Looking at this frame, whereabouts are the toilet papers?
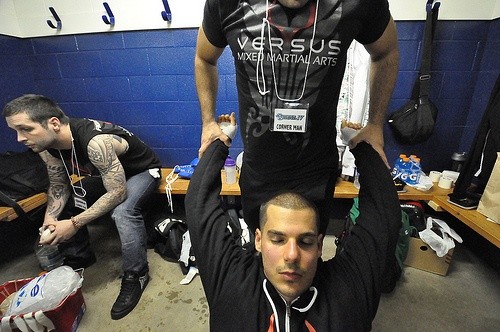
[428,171,441,182]
[438,177,453,189]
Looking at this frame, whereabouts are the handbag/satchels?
[148,212,187,262]
[477,151,500,224]
[0,149,50,203]
[388,95,438,145]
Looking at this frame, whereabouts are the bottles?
[394,154,421,184]
[224,155,236,184]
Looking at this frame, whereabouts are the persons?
[195,1,400,234]
[184,111,400,332]
[3,95,162,319]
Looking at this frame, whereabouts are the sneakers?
[62,253,97,272]
[110,264,150,320]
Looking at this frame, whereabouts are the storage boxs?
[0,277,88,332]
[403,237,456,277]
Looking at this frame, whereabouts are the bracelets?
[71,216,80,230]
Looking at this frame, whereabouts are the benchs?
[432,194,500,248]
[154,168,242,211]
[0,173,86,222]
[332,175,456,200]
